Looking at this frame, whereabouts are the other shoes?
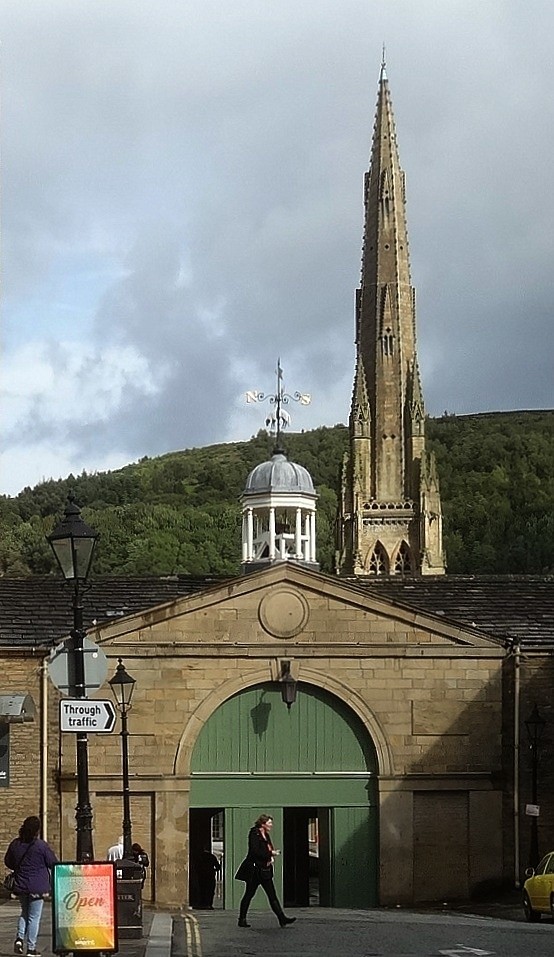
[208,906,214,910]
[279,917,296,928]
[202,906,207,910]
[237,918,251,928]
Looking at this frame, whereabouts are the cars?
[521,852,554,922]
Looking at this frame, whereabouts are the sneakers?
[14,940,23,954]
[26,949,41,957]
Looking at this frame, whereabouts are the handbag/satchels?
[245,856,267,886]
[4,875,15,891]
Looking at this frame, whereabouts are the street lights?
[107,657,139,862]
[45,494,101,865]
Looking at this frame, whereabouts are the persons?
[131,842,149,890]
[4,816,61,957]
[233,815,297,928]
[195,845,221,910]
[107,835,124,879]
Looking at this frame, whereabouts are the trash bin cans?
[116,857,143,938]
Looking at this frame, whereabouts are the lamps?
[280,660,297,711]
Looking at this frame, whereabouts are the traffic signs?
[60,698,116,734]
